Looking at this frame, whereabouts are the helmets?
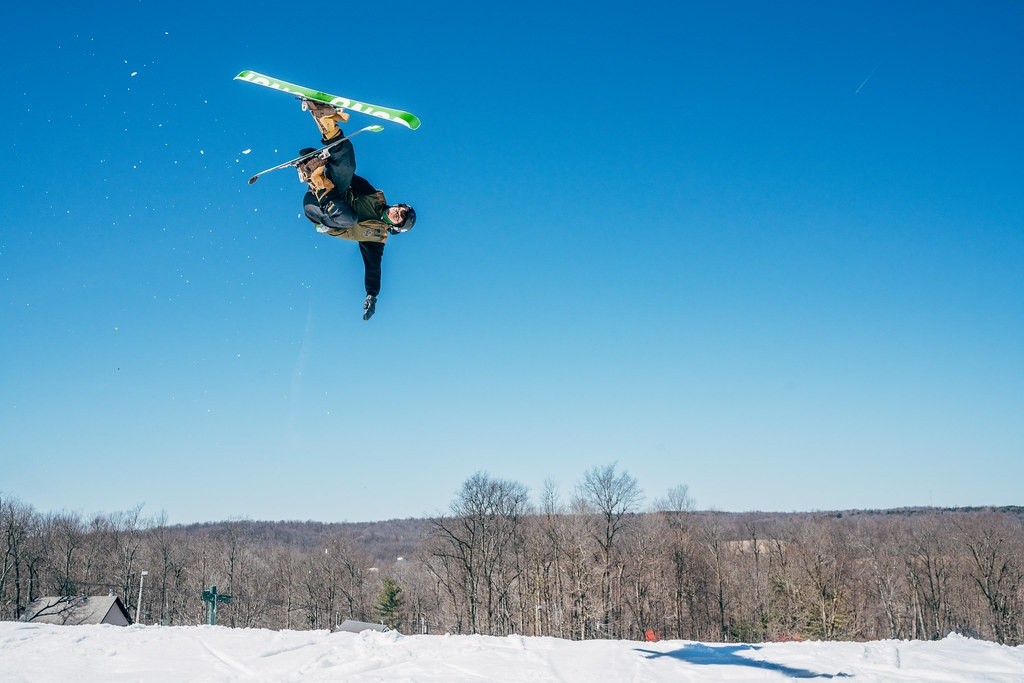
[388,203,416,233]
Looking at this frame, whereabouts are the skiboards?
[232,71,421,185]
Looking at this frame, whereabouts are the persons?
[293,98,417,320]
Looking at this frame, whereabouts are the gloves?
[300,148,317,155]
[363,295,377,321]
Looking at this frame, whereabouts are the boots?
[302,159,335,201]
[309,109,350,140]
